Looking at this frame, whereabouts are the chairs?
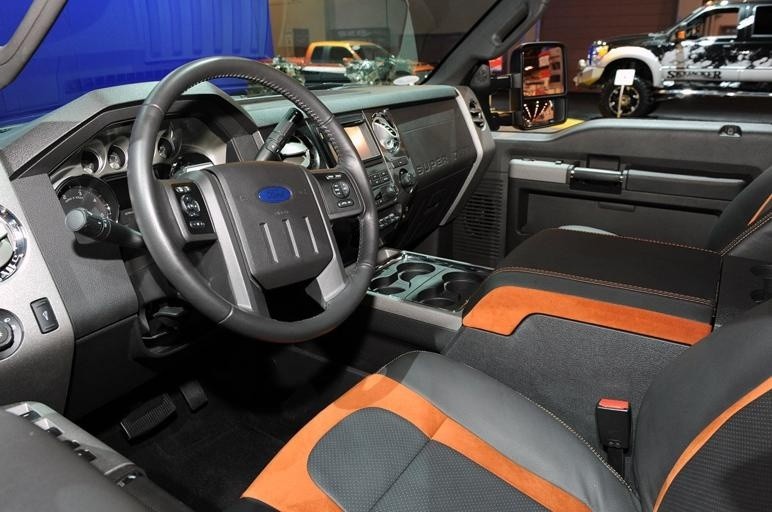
[219,298,771,511]
[462,173,771,347]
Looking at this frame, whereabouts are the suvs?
[572,0,772,118]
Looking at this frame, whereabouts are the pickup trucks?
[254,40,435,85]
